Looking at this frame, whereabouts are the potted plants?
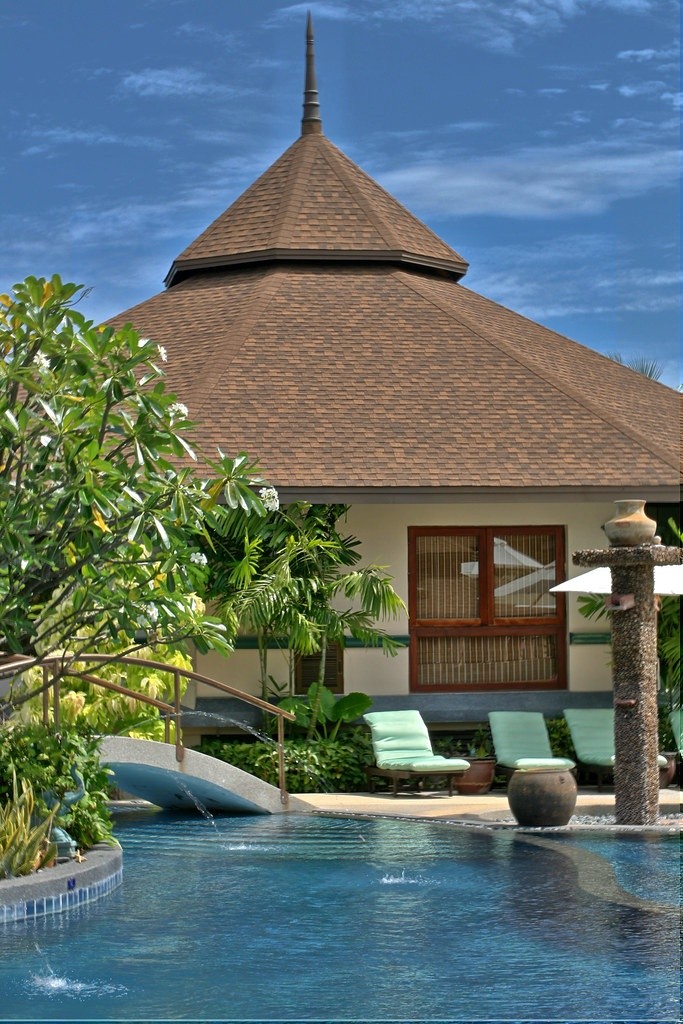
[449,744,497,795]
[658,752,677,789]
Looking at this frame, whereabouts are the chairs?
[353,709,471,797]
[488,711,576,793]
[563,708,667,792]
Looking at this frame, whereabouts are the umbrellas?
[547,548,683,596]
[460,535,557,607]
